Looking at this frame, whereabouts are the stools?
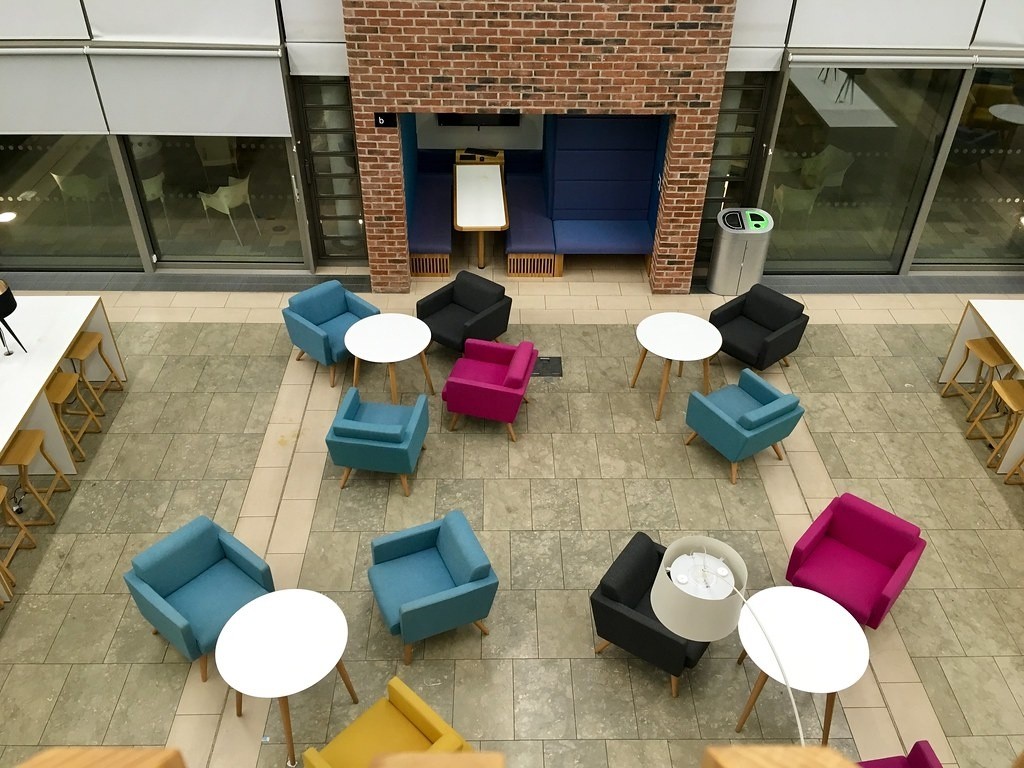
[47,330,124,462]
[777,88,820,148]
[0,429,72,587]
[941,337,1024,486]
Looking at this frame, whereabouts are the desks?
[0,296,127,601]
[769,148,804,175]
[631,312,723,420]
[790,68,899,205]
[103,137,162,160]
[344,313,435,403]
[736,585,869,746]
[215,588,358,766]
[937,299,1024,475]
[986,103,1024,172]
[453,163,509,268]
[735,585,870,746]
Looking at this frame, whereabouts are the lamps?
[651,536,806,747]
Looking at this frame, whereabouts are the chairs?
[590,531,710,698]
[122,515,275,681]
[961,83,1020,140]
[302,675,474,768]
[282,280,380,386]
[708,283,809,373]
[141,172,172,236]
[685,368,804,483]
[769,181,825,229]
[442,337,539,441]
[786,493,927,632]
[857,740,944,768]
[416,269,512,357]
[194,136,240,192]
[325,387,430,497]
[798,144,856,206]
[199,172,262,247]
[50,171,115,226]
[367,507,500,666]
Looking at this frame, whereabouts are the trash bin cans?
[705,207,775,296]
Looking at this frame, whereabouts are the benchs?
[552,220,654,278]
[506,174,554,277]
[408,174,452,277]
